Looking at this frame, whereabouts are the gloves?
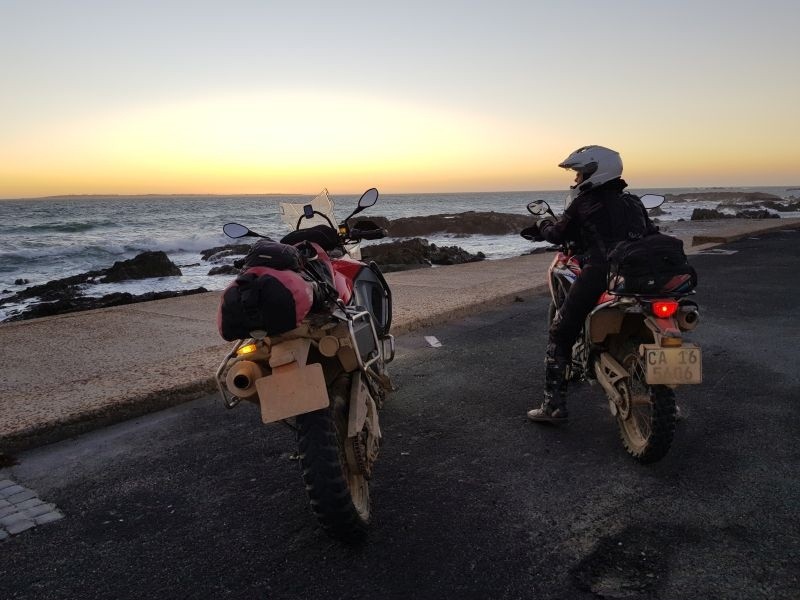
[520,219,554,242]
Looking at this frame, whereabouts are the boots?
[675,405,680,421]
[527,356,572,423]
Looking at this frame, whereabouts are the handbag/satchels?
[217,240,335,342]
[605,220,698,293]
[245,238,305,272]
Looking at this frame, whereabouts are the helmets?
[558,145,623,202]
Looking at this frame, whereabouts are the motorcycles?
[216,187,398,533]
[524,194,702,463]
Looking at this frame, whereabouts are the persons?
[528,145,660,422]
